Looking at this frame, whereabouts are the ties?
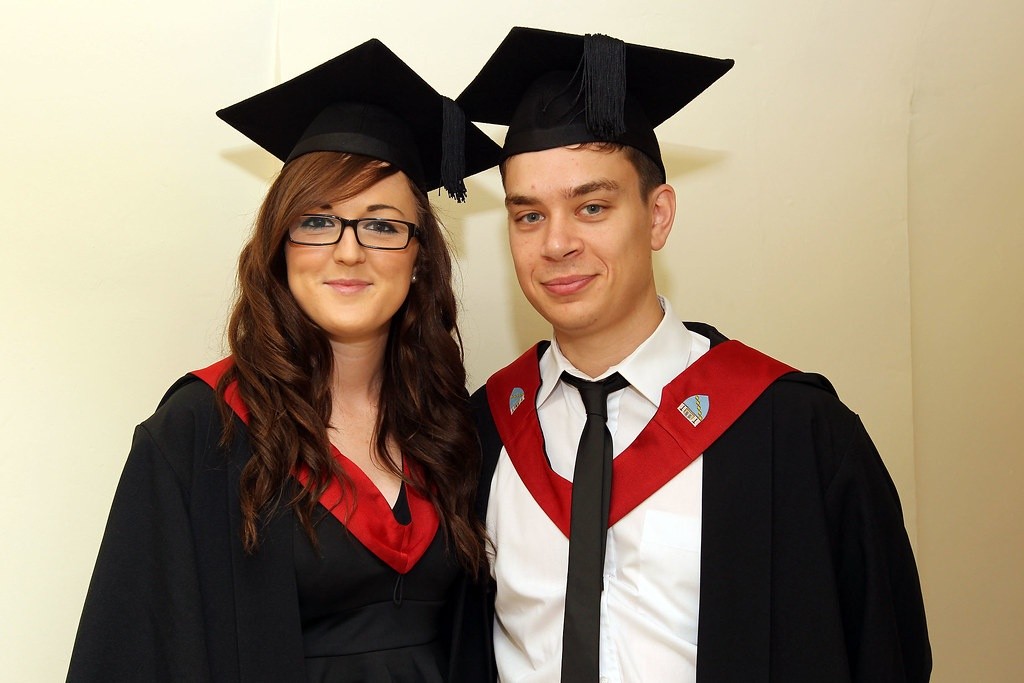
[560,370,631,683]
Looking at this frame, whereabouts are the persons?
[66,36,503,683]
[454,26,932,683]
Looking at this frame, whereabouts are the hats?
[456,26,735,185]
[216,38,503,199]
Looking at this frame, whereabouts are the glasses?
[287,214,425,250]
